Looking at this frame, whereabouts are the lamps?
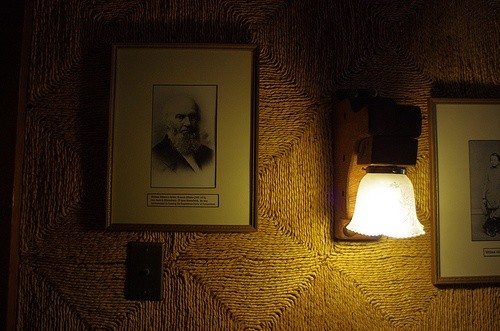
[331,88,426,247]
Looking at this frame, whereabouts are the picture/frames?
[102,40,262,234]
[426,94,500,289]
